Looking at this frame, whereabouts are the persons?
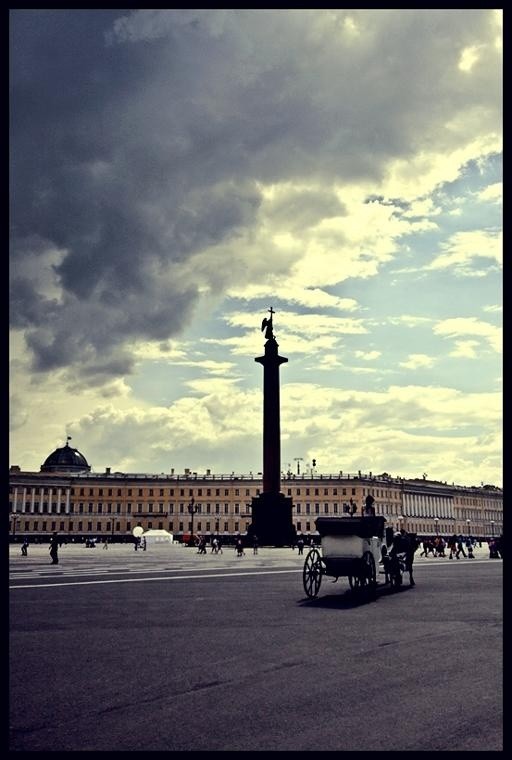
[188,532,224,554]
[21,536,29,556]
[252,535,259,554]
[49,532,58,564]
[235,534,243,556]
[361,495,375,517]
[102,539,108,550]
[298,536,304,555]
[380,526,503,587]
[142,536,146,551]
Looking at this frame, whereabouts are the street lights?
[187,498,199,549]
[396,515,403,532]
[345,503,357,517]
[465,519,471,538]
[11,511,21,542]
[434,517,439,538]
[489,520,494,540]
[110,517,117,536]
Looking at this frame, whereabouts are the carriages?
[298,515,421,596]
